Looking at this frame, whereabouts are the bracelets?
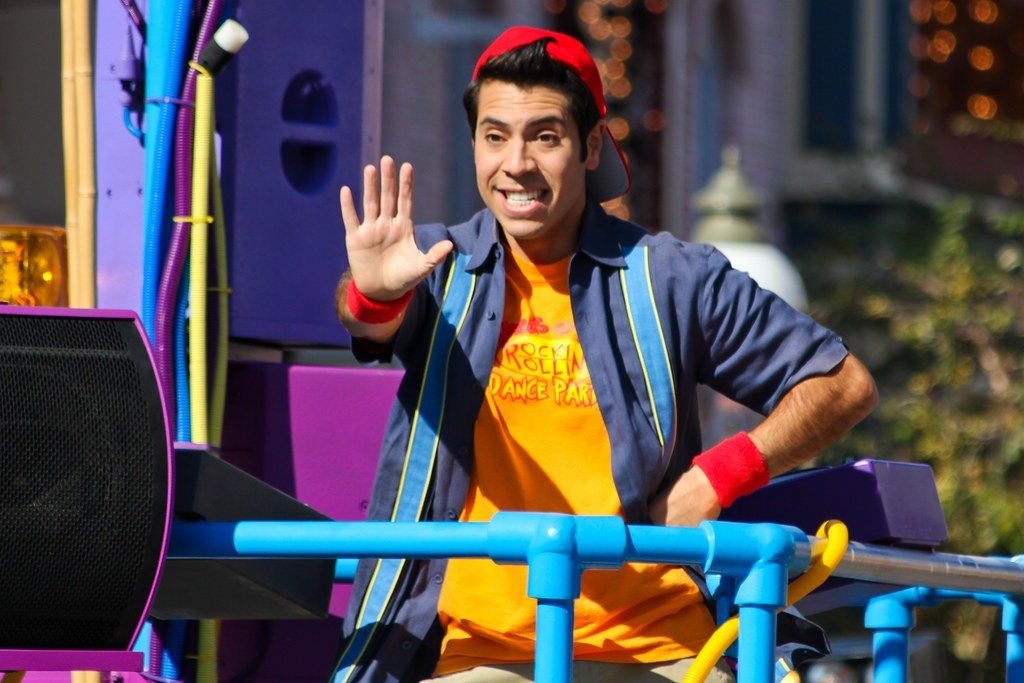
[345,278,414,324]
[689,432,770,508]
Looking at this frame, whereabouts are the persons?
[323,27,878,683]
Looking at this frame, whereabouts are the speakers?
[0,303,176,659]
[206,0,365,353]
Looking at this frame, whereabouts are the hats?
[467,26,632,204]
